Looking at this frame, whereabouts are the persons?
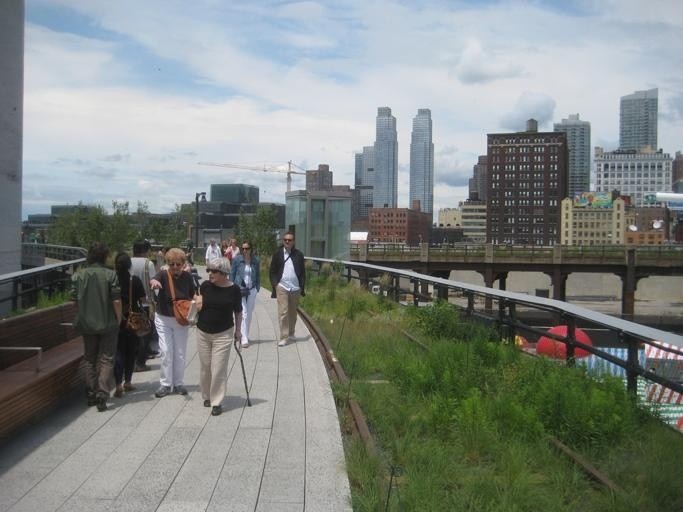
[21,229,48,244]
[129,238,161,359]
[269,233,304,346]
[112,252,147,397]
[71,243,123,412]
[149,235,263,416]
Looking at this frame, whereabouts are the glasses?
[206,268,221,273]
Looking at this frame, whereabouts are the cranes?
[196,159,305,192]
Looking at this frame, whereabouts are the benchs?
[0,305,86,442]
[60,301,85,343]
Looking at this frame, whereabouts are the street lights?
[194,192,208,248]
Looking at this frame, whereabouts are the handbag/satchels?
[173,300,199,326]
[125,311,151,337]
[149,299,156,320]
[240,287,249,297]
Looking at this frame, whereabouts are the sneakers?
[154,386,171,398]
[278,336,296,346]
[203,401,221,415]
[87,384,135,411]
[173,386,187,395]
